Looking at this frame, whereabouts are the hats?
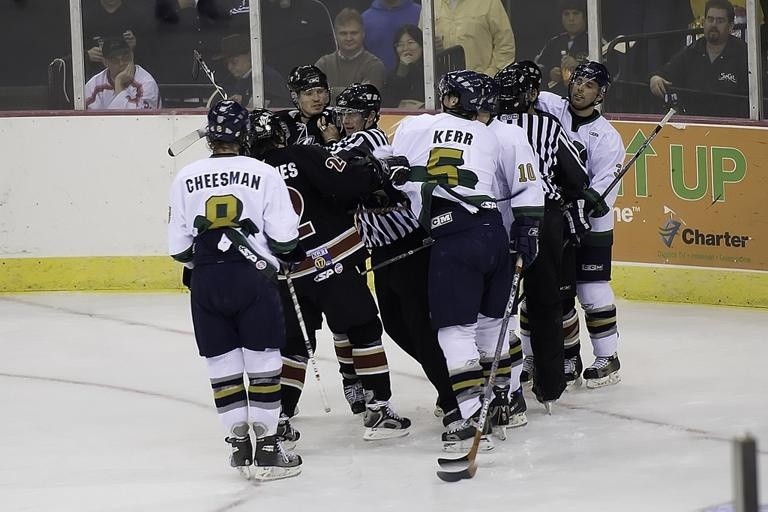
[211,33,251,62]
[102,37,131,57]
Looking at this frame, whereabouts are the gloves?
[560,198,593,249]
[378,155,412,186]
[509,216,541,271]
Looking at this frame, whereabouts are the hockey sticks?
[437,254,524,482]
[192,50,231,100]
[168,128,210,156]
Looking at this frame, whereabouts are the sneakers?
[583,352,622,380]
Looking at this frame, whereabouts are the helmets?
[335,83,383,130]
[207,100,290,144]
[438,59,544,115]
[285,64,332,119]
[568,60,611,111]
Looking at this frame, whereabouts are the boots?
[442,351,583,442]
[343,374,367,416]
[276,419,301,441]
[225,433,254,467]
[363,404,412,430]
[254,434,303,468]
